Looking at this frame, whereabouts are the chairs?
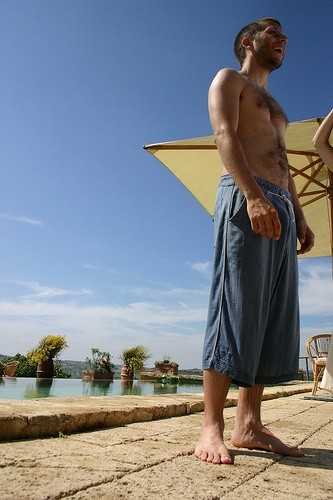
[307,333,333,395]
[4,360,20,377]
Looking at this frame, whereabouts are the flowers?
[79,347,115,373]
[25,333,70,366]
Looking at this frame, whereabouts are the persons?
[311,108,333,173]
[193,18,316,464]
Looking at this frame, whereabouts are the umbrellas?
[143,117,333,259]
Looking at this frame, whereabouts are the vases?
[36,359,55,378]
[81,370,115,381]
[35,378,54,390]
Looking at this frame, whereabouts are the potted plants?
[116,344,153,381]
[120,380,141,396]
[154,355,179,376]
[154,383,177,394]
